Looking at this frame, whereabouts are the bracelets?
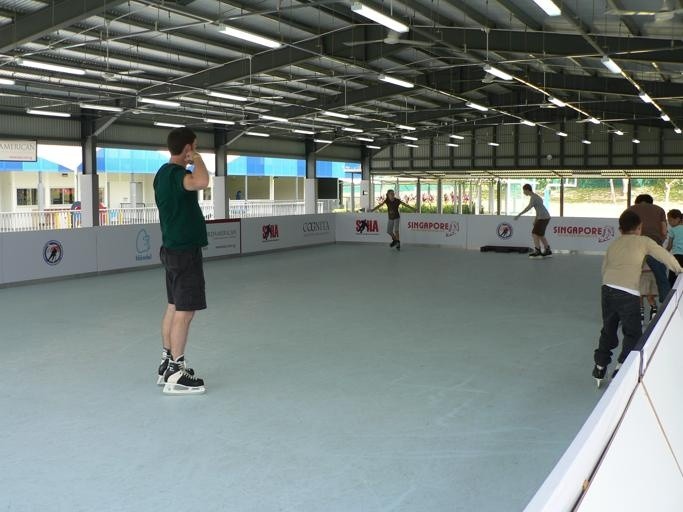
[191,152,201,162]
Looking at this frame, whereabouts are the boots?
[157,346,195,377]
[639,306,645,320]
[540,245,553,256]
[162,353,204,387]
[590,351,610,381]
[609,358,622,377]
[648,304,658,320]
[528,246,542,257]
[389,238,400,248]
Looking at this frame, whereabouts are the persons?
[593,210,682,387]
[367,190,419,250]
[512,184,553,259]
[638,259,659,327]
[151,127,210,395]
[622,193,671,304]
[665,209,682,290]
